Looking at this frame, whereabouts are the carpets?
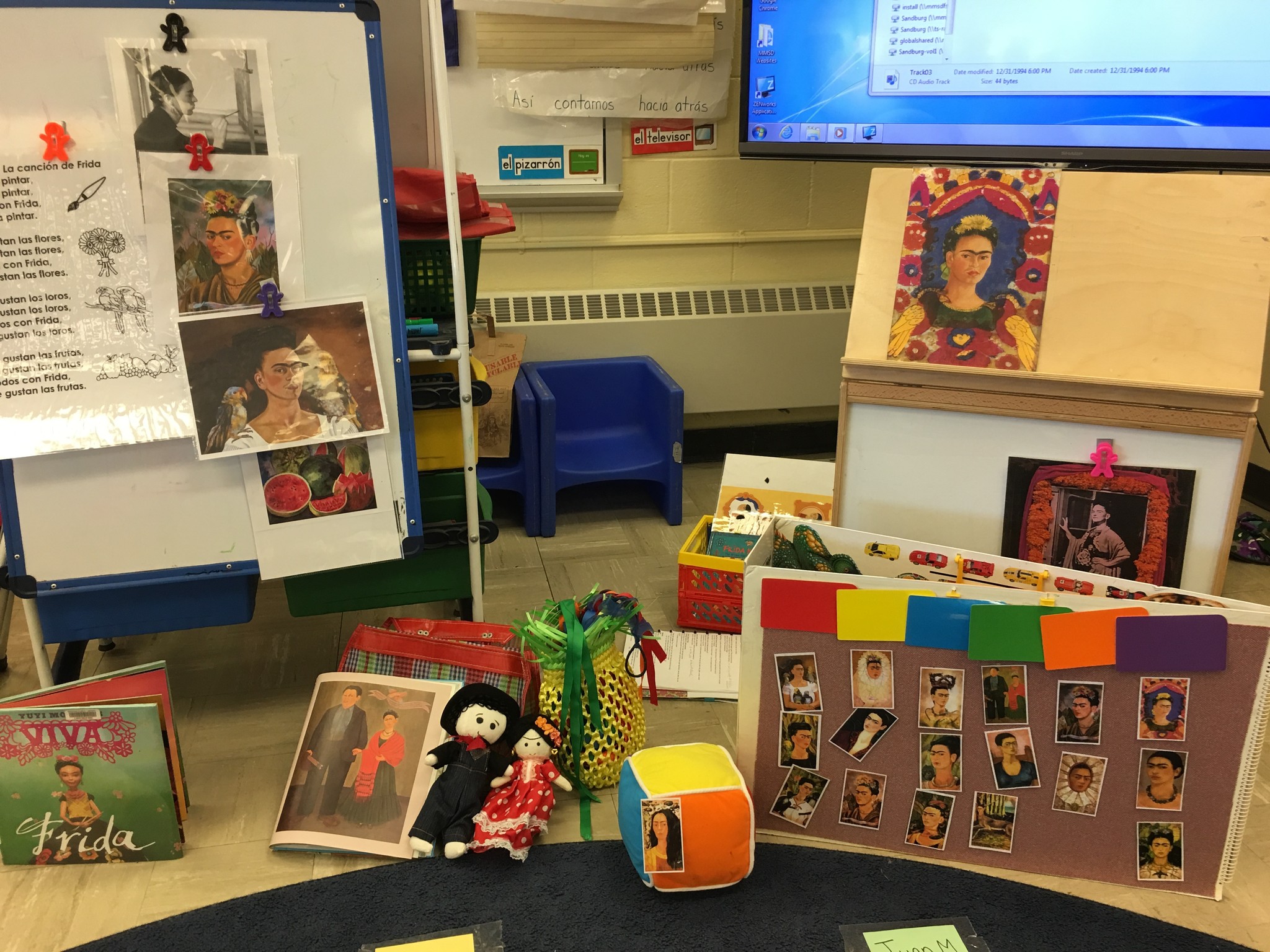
[68,840,1259,952]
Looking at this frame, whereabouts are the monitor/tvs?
[741,0,1270,173]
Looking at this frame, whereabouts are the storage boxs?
[467,327,528,459]
[36,574,258,643]
[677,514,745,630]
[283,234,486,618]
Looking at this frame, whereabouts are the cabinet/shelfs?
[0,315,497,689]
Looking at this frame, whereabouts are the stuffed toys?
[408,683,521,859]
[464,714,573,863]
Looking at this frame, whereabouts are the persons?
[134,65,229,155]
[1059,501,1131,577]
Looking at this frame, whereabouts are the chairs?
[519,356,684,538]
[478,367,540,536]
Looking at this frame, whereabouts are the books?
[0,658,190,865]
[270,673,466,859]
[692,531,760,625]
